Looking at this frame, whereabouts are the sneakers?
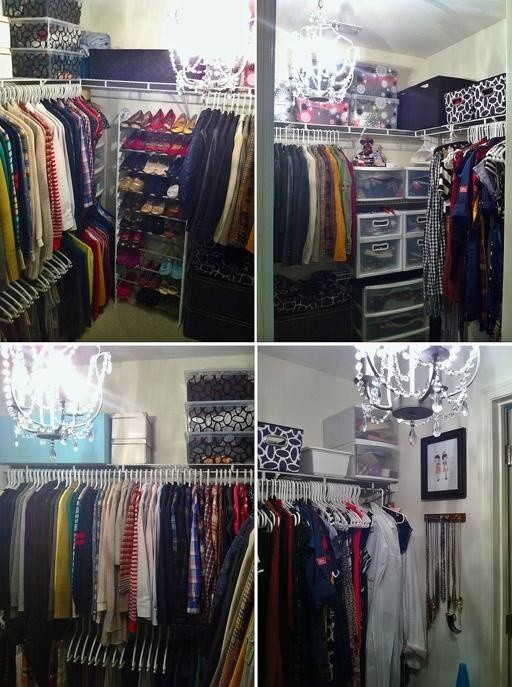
[117,108,200,313]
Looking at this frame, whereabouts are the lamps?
[1,343,114,459]
[348,344,483,446]
[284,0,359,108]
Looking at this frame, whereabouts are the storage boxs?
[286,49,506,128]
[0,413,154,467]
[318,405,401,480]
[1,0,85,80]
[257,421,353,476]
[350,159,437,343]
[181,366,255,466]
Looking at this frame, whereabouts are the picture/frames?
[416,427,469,501]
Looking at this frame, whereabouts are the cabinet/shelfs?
[187,241,255,339]
[104,110,200,334]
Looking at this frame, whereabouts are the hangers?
[257,475,410,531]
[202,90,254,126]
[1,82,89,108]
[273,125,346,151]
[0,250,76,327]
[67,612,172,674]
[1,465,255,495]
[454,120,505,145]
